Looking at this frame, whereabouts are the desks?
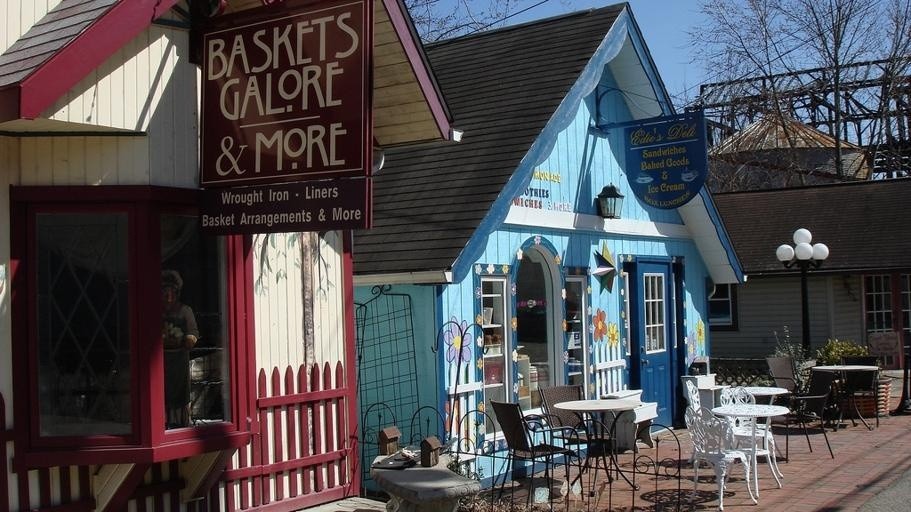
[553,399,641,491]
[711,385,793,501]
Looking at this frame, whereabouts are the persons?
[157,267,201,430]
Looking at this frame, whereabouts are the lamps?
[597,181,625,219]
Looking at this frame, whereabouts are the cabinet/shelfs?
[481,274,511,441]
[566,276,590,432]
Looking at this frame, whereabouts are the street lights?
[775,228,830,360]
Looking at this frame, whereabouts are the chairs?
[490,382,619,508]
[766,353,882,458]
[685,379,785,510]
